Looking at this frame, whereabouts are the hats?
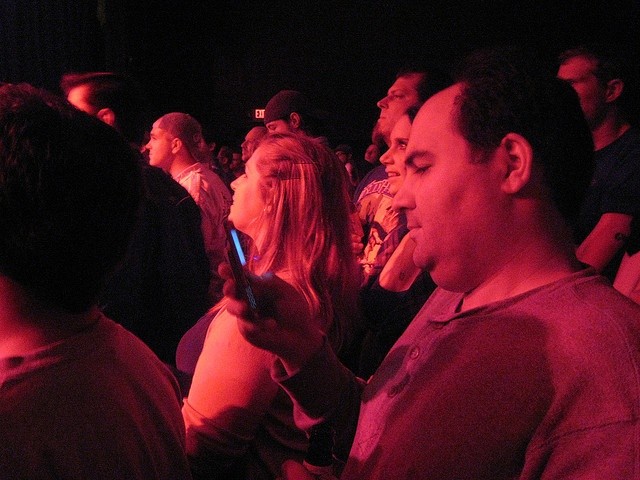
[152,112,208,164]
[264,88,316,124]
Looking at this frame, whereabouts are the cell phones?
[222,221,261,319]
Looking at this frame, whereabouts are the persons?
[60,69,211,361]
[335,143,362,187]
[214,47,640,479]
[377,103,425,195]
[351,66,447,357]
[200,122,219,175]
[239,125,267,161]
[363,143,377,163]
[231,150,245,177]
[216,144,235,179]
[0,77,189,480]
[176,125,358,479]
[140,110,232,298]
[263,88,361,277]
[553,31,640,270]
[610,213,640,303]
